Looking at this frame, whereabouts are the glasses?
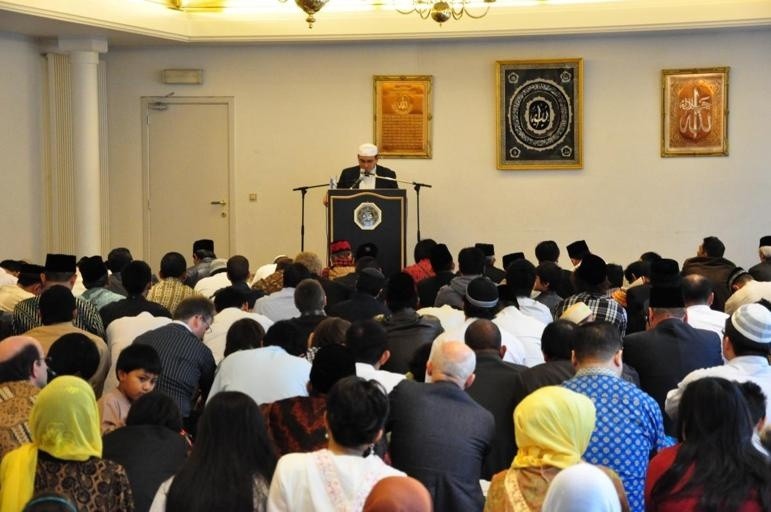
[196,313,212,334]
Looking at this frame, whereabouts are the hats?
[430,244,452,269]
[19,253,105,279]
[194,239,214,251]
[559,301,592,326]
[358,143,378,157]
[476,244,494,256]
[209,258,227,274]
[503,252,524,268]
[465,278,499,309]
[567,240,588,257]
[330,240,350,254]
[650,258,685,307]
[727,265,748,295]
[498,284,516,300]
[760,236,771,246]
[731,303,771,344]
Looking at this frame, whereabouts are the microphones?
[359,167,374,178]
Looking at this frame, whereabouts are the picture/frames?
[495,58,584,170]
[373,75,434,160]
[661,66,730,157]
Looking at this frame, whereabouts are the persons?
[0,237,771,512]
[323,143,399,207]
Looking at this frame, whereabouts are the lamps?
[250,0,373,30]
[388,0,496,28]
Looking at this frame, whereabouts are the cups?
[329,175,338,189]
